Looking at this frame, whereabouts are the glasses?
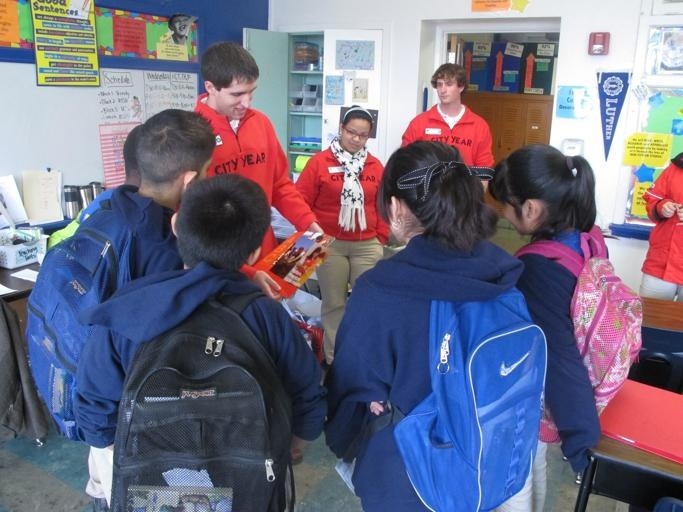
[342,126,369,141]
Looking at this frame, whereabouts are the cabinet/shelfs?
[239,25,390,180]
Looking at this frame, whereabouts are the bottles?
[64,181,105,221]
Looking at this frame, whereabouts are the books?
[288,136,321,153]
[253,227,336,298]
[126,483,233,512]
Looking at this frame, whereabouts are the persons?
[165,13,191,45]
[294,104,391,389]
[488,143,608,511]
[79,123,144,222]
[400,63,496,193]
[69,172,328,512]
[321,140,525,511]
[87,108,215,510]
[192,41,326,325]
[272,246,327,288]
[637,152,683,302]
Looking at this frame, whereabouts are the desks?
[552,293,683,511]
[0,261,46,448]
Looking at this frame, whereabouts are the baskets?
[0,234,50,269]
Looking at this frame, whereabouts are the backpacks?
[512,224,644,443]
[107,288,295,511]
[24,198,139,442]
[392,283,546,512]
